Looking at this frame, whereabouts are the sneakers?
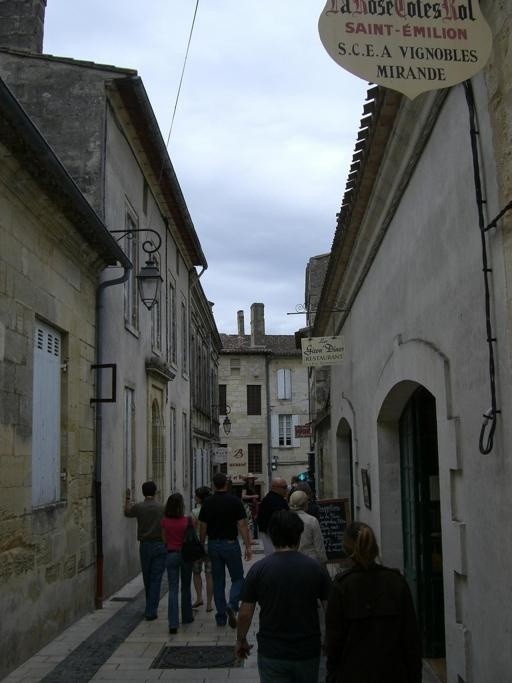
[207,608,213,612]
[193,600,204,608]
[226,607,236,628]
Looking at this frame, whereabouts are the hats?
[289,490,310,510]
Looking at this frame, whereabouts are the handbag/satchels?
[181,516,206,563]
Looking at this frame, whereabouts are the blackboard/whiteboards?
[316,497,352,563]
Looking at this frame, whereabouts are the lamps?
[111,226,165,312]
[212,403,232,435]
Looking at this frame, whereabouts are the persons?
[321,520,426,682]
[232,508,340,683]
[122,466,330,635]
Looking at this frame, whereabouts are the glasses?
[273,485,288,490]
